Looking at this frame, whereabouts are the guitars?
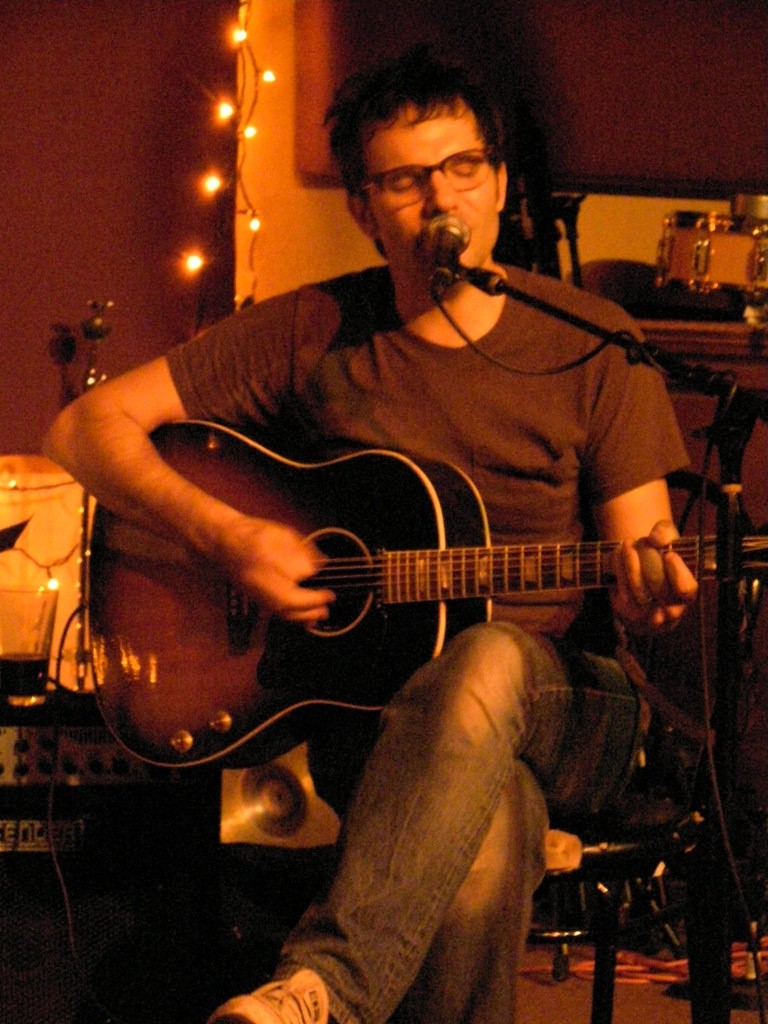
[86,416,768,778]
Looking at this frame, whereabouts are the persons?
[43,43,698,1024]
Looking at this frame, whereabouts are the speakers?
[0,651,266,1024]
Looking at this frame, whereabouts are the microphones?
[417,213,472,302]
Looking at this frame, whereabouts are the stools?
[542,795,726,1024]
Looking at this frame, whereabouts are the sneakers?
[207,966,329,1023]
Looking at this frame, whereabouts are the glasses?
[359,144,493,208]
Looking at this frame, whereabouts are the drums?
[531,840,656,940]
[652,210,767,302]
